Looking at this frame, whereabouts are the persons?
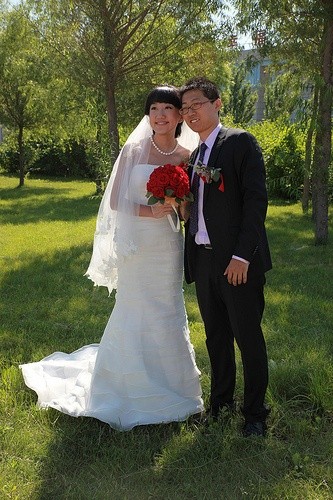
[178,76,271,436]
[18,85,206,431]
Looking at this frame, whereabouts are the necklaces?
[150,136,178,155]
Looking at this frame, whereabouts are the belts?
[195,244,213,251]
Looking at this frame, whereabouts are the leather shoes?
[202,416,231,429]
[245,416,267,437]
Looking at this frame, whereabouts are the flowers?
[193,160,221,184]
[144,161,194,221]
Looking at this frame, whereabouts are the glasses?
[179,99,214,115]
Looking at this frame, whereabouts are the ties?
[189,143,208,236]
